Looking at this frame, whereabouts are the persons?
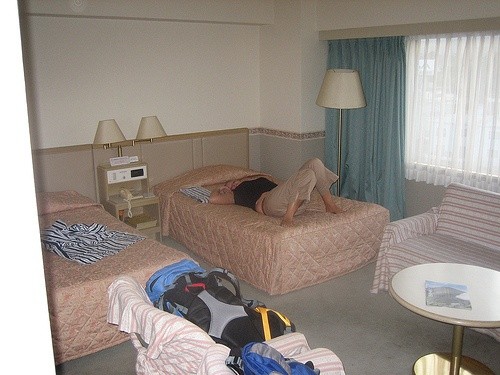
[209,157,344,227]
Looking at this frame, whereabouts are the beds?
[36,190,200,368]
[149,165,390,296]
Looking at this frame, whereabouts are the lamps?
[92,119,126,149]
[132,116,167,146]
[315,68,368,197]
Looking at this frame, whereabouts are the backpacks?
[188,267,297,354]
[145,258,265,317]
[224,340,321,375]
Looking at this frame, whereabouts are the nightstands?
[97,161,163,242]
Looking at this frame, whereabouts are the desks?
[389,261,500,375]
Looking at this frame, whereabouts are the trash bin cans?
[412,352,496,375]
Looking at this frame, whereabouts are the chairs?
[105,276,311,375]
[195,342,346,375]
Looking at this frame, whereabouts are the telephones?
[120,188,143,199]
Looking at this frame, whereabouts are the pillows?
[36,191,80,216]
[181,163,260,188]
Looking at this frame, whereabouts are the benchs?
[370,182,500,343]
